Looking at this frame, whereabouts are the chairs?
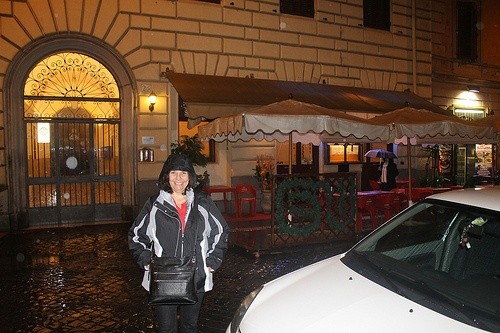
[450,232,500,277]
[234,183,256,218]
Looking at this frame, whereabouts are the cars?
[224,187,500,333]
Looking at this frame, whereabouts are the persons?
[378,158,398,192]
[127,156,231,333]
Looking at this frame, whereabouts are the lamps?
[148,91,157,111]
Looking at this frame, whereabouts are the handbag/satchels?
[149,256,198,305]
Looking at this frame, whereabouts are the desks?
[205,185,237,219]
[323,178,462,232]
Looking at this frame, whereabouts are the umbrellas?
[364,150,398,160]
[200,97,498,204]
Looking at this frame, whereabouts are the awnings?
[165,73,448,122]
